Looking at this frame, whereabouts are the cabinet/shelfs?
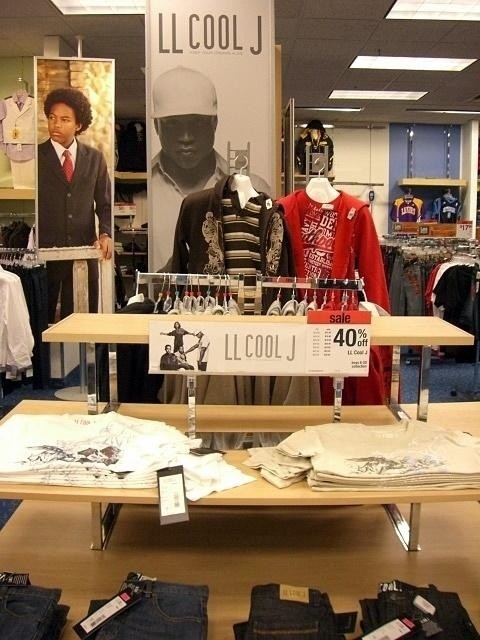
[0,312,479,640]
[114,170,148,280]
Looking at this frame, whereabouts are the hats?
[151,66,218,119]
[194,329,200,336]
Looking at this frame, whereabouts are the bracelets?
[98,232,108,238]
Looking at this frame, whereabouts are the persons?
[157,176,321,405]
[39,88,113,324]
[152,67,273,272]
[160,322,196,353]
[160,345,178,370]
[185,330,209,371]
[174,346,194,370]
[275,176,393,405]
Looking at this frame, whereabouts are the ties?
[62,150,74,182]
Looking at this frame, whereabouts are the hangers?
[230,154,256,208]
[15,78,28,102]
[154,272,382,317]
[131,271,142,293]
[306,158,339,199]
[404,186,415,200]
[444,187,452,199]
[445,251,480,269]
[0,210,37,235]
